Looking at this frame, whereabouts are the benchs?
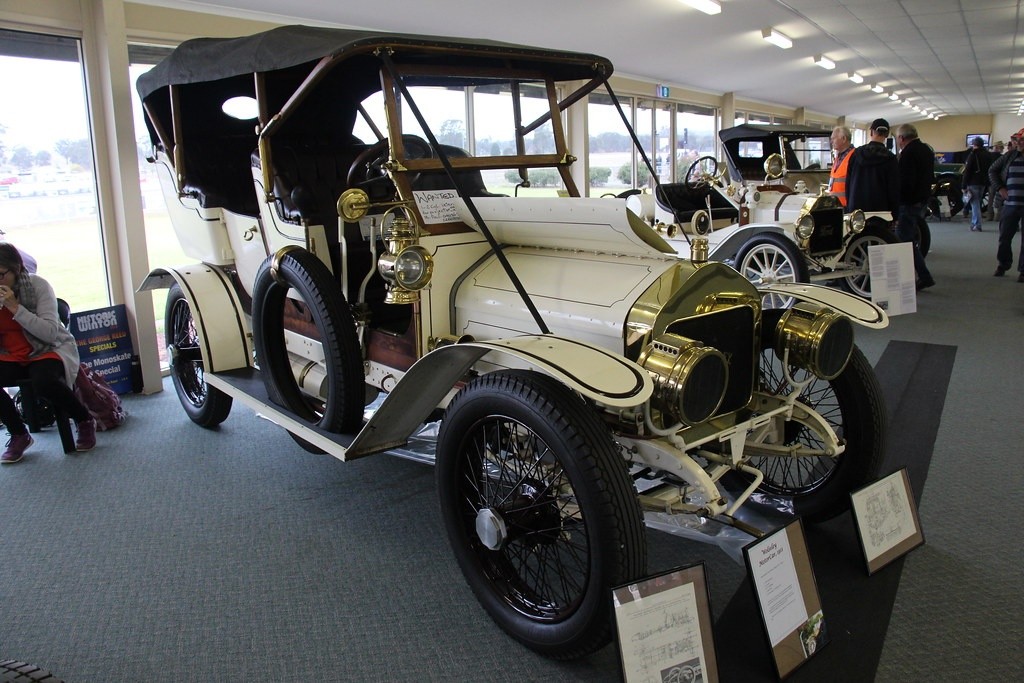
[184,134,363,215]
[656,181,739,223]
[735,158,784,180]
[257,144,486,265]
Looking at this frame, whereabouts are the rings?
[4,292,7,294]
[1,294,3,296]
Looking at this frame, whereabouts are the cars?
[928,164,990,216]
[136,24,890,660]
[601,156,901,310]
[716,122,930,260]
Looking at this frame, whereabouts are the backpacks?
[76,363,127,431]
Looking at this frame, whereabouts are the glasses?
[0,269,11,282]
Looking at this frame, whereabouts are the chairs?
[0,298,75,454]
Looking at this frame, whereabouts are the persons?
[844,118,936,292]
[0,242,97,463]
[962,128,1024,283]
[828,126,855,214]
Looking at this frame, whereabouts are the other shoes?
[969,224,982,232]
[1017,271,1024,283]
[994,261,1008,276]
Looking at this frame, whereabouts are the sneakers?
[916,279,936,290]
[0,432,35,464]
[75,418,97,452]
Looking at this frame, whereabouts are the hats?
[869,118,890,134]
[993,141,1005,147]
[1011,128,1024,138]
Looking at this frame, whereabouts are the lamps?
[679,0,721,15]
[848,72,864,84]
[871,83,883,93]
[887,91,899,100]
[761,27,793,49]
[901,99,939,120]
[814,54,835,69]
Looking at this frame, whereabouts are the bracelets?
[12,314,16,320]
